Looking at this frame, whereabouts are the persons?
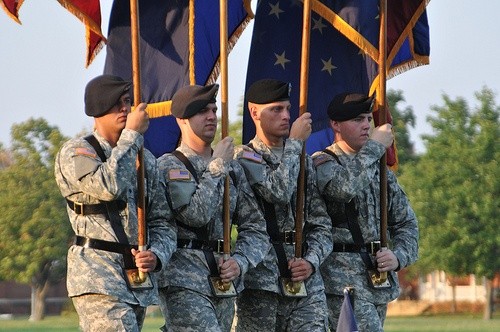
[55,75,176,332]
[311,94,420,332]
[157,84,271,332]
[236,78,333,332]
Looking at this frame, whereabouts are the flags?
[0,0,24,24]
[56,0,108,68]
[103,0,430,172]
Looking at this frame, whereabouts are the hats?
[171,83,219,119]
[327,93,377,122]
[85,74,133,117]
[247,79,292,104]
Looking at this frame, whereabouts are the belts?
[76,236,149,256]
[177,238,232,254]
[333,241,381,255]
[269,231,305,245]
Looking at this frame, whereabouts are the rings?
[381,262,384,267]
